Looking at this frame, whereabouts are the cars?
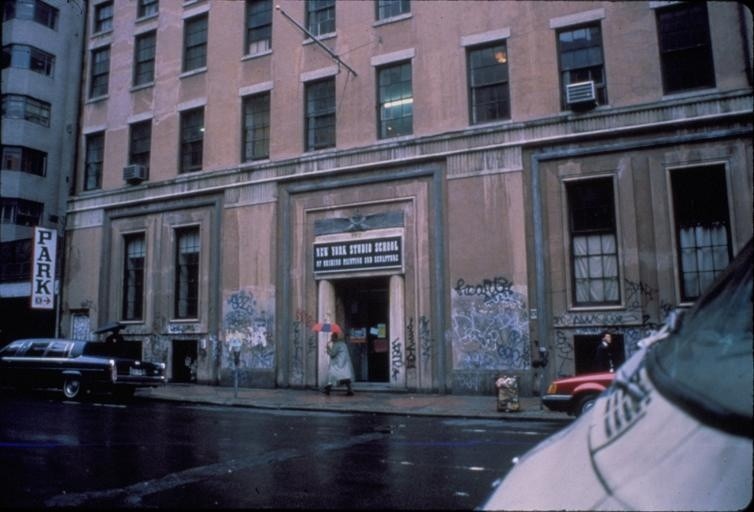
[542,372,617,419]
[478,233,754,512]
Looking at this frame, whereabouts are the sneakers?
[322,390,354,395]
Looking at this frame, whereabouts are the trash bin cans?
[496,376,519,411]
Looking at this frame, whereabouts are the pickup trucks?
[0,338,168,402]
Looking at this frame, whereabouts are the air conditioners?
[123,164,147,180]
[566,80,597,105]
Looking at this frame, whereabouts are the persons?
[595,332,619,372]
[320,332,356,395]
[105,326,127,358]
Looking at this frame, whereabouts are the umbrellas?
[311,322,342,351]
[92,321,126,334]
